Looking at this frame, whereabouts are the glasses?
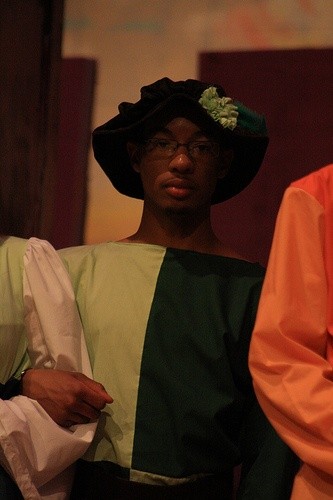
[142,136,221,164]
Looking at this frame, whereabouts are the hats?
[92,76,267,204]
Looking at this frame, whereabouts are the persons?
[1,231,98,500]
[246,158,333,500]
[16,75,307,500]
